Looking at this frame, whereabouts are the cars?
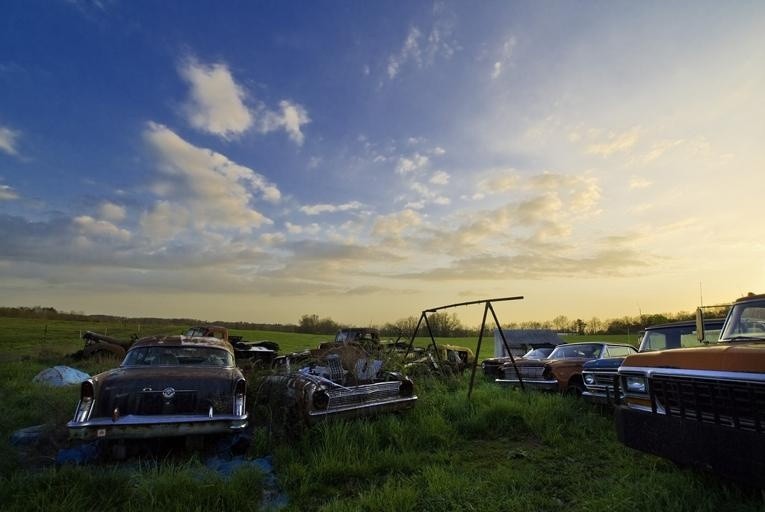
[478,346,557,379]
[492,341,638,397]
[616,291,765,438]
[65,334,252,443]
[633,316,765,356]
[578,355,629,407]
